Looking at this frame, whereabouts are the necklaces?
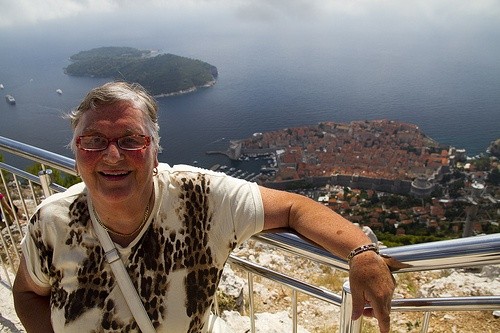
[91,189,153,237]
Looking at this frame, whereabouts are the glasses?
[76,133,153,152]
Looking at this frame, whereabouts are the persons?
[11,80,397,333]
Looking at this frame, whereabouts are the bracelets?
[345,243,379,262]
